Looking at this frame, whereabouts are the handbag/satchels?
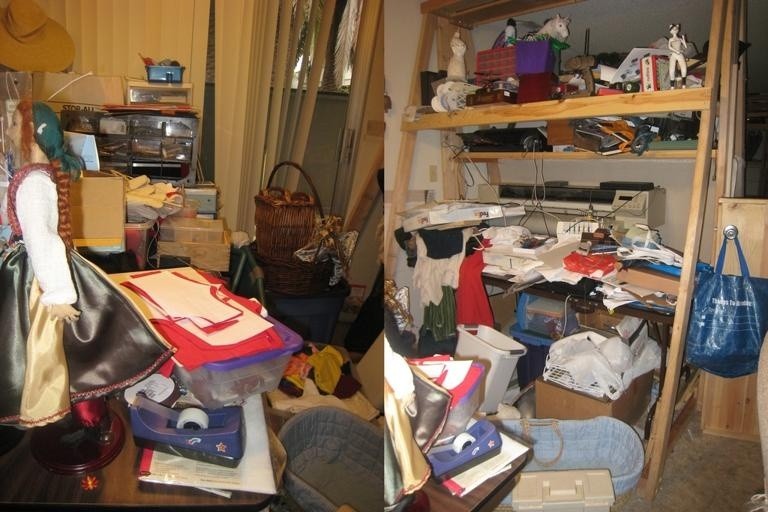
[684,237,768,377]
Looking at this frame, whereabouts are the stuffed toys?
[529,13,573,43]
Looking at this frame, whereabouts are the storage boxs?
[68,171,231,272]
[266,271,350,344]
[123,65,194,105]
[508,294,660,428]
[171,309,304,411]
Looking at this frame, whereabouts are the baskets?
[255,188,314,263]
[268,260,331,296]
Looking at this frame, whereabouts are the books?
[137,395,278,498]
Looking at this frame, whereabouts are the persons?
[0,99,179,476]
[667,22,692,87]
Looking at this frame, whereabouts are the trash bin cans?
[456,322,528,416]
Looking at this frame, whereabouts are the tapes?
[132,397,210,431]
[427,433,477,458]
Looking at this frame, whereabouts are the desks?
[0,269,282,511]
[408,257,691,440]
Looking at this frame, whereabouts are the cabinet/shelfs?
[385,0,748,501]
[701,197,767,443]
